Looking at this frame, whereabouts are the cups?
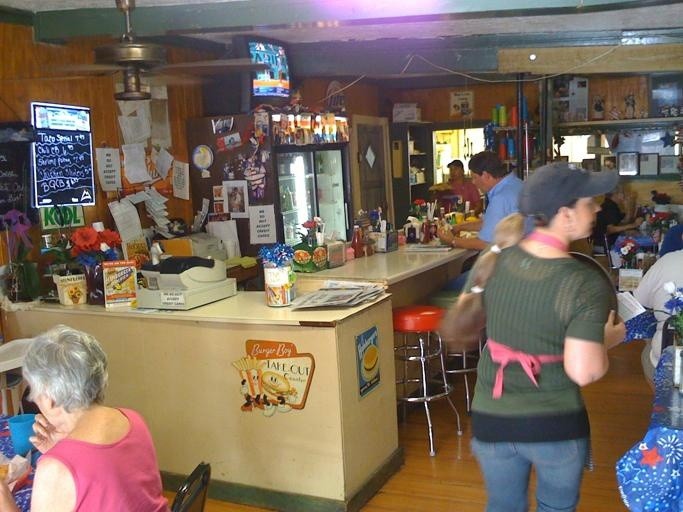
[498,136,515,159]
[8,413,40,456]
[263,266,290,307]
[362,240,374,256]
[491,103,518,128]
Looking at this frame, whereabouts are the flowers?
[294,220,315,247]
[409,199,426,221]
[61,226,122,269]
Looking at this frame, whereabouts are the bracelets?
[452,239,456,248]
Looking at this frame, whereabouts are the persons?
[433,159,483,216]
[442,162,620,512]
[229,187,242,212]
[0,324,170,512]
[437,151,525,251]
[594,189,644,257]
[632,248,683,393]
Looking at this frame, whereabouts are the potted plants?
[39,239,90,308]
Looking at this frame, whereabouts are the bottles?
[352,225,363,257]
[278,150,325,213]
[419,207,458,244]
[283,216,305,241]
[407,225,415,242]
[345,241,354,261]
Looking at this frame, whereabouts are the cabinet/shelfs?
[489,124,536,174]
[386,119,434,229]
[553,116,682,182]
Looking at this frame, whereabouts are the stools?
[427,289,484,415]
[390,302,463,458]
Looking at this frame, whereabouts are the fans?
[1,1,272,107]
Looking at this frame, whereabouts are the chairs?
[169,459,211,511]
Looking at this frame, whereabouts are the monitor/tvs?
[202,34,294,115]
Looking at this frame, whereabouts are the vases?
[78,259,104,305]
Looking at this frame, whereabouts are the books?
[406,244,452,252]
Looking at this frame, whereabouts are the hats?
[519,161,620,226]
[448,160,463,168]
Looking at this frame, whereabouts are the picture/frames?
[555,151,681,176]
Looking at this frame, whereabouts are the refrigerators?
[183,111,355,259]
